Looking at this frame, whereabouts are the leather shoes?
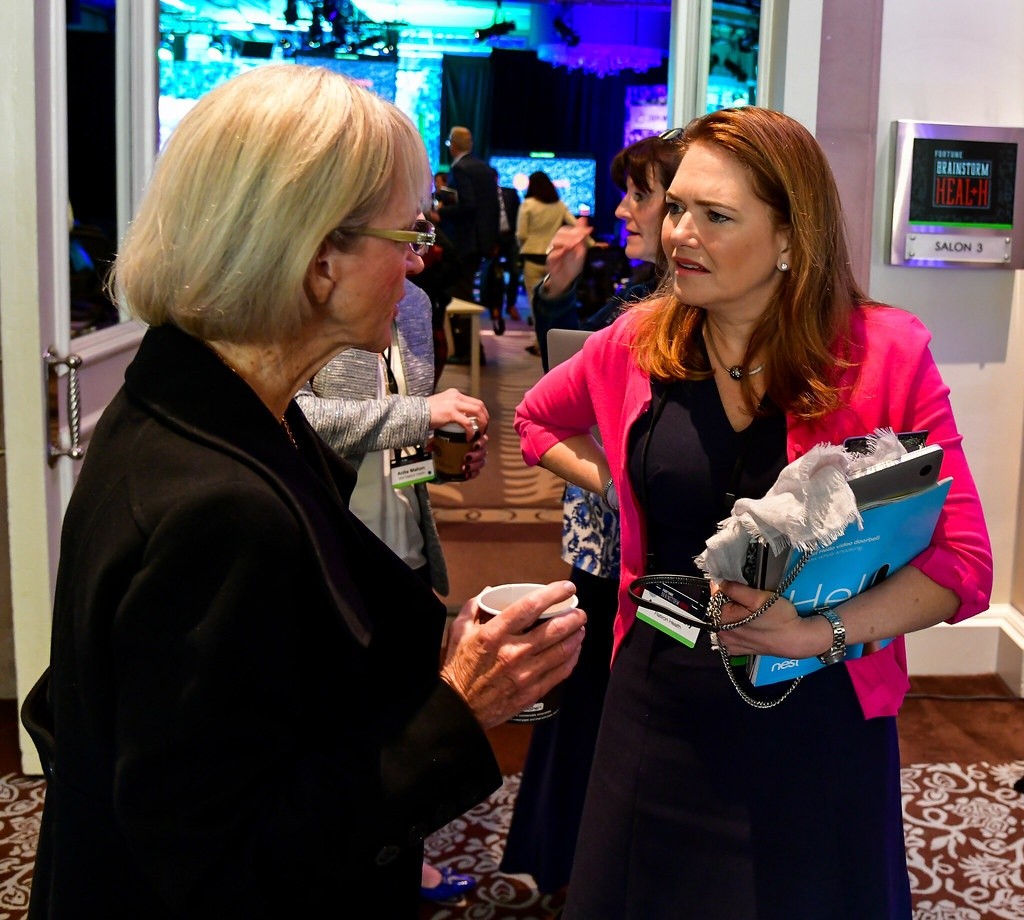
[420,870,475,898]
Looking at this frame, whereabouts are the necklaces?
[702,314,767,378]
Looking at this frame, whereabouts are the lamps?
[473,21,516,42]
[552,16,581,49]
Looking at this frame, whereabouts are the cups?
[432,415,481,480]
[477,582,579,723]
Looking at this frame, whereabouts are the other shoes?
[493,311,504,334]
[507,308,521,320]
[528,316,534,326]
[525,345,539,354]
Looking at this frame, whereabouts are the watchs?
[814,606,846,664]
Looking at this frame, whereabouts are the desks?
[444,298,485,400]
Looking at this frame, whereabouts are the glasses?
[338,220,436,254]
[660,128,685,140]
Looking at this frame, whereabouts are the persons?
[293,281,489,903]
[20,58,588,920]
[515,107,995,920]
[405,171,460,396]
[491,168,522,324]
[496,128,703,919]
[516,169,608,354]
[447,128,502,366]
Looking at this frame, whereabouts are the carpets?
[0,761,1024,920]
